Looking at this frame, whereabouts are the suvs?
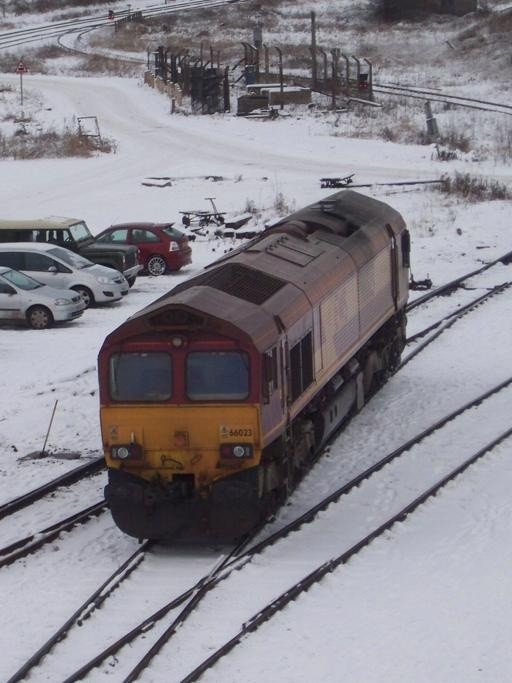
[1,216,144,287]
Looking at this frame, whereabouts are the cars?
[0,241,129,308]
[94,224,193,277]
[1,267,86,330]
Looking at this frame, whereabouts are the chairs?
[133,230,147,241]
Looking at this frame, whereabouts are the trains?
[97,189,411,542]
